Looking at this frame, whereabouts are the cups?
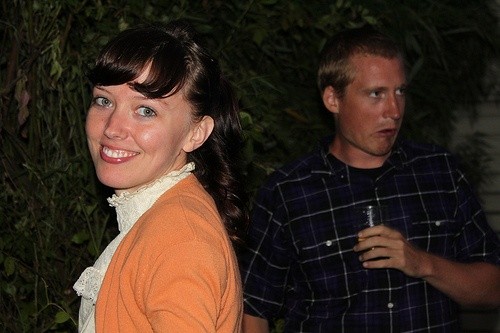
[349,206,383,262]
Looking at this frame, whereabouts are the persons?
[232,30,499,333]
[63,23,246,333]
